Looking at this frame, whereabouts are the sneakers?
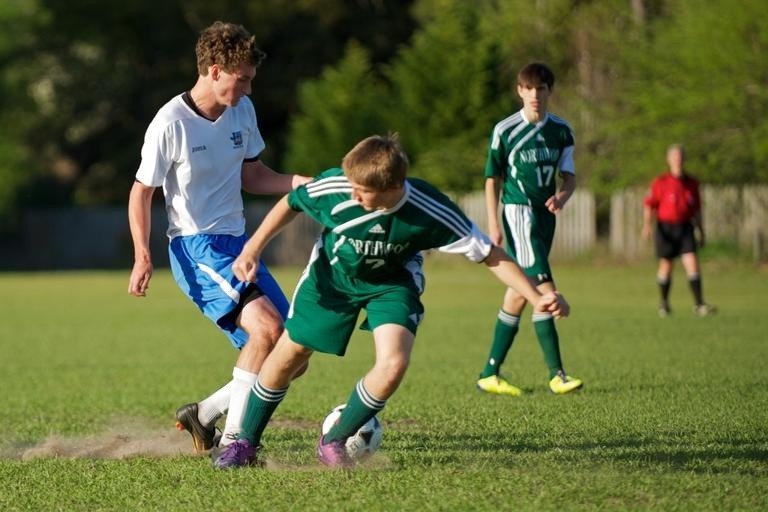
[317,433,356,472]
[546,371,583,395]
[476,372,525,399]
[176,403,268,474]
[659,299,670,316]
[692,304,717,319]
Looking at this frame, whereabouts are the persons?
[212,135,571,469]
[128,22,315,461]
[479,62,583,396]
[643,144,717,317]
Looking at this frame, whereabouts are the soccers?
[323,403,380,463]
